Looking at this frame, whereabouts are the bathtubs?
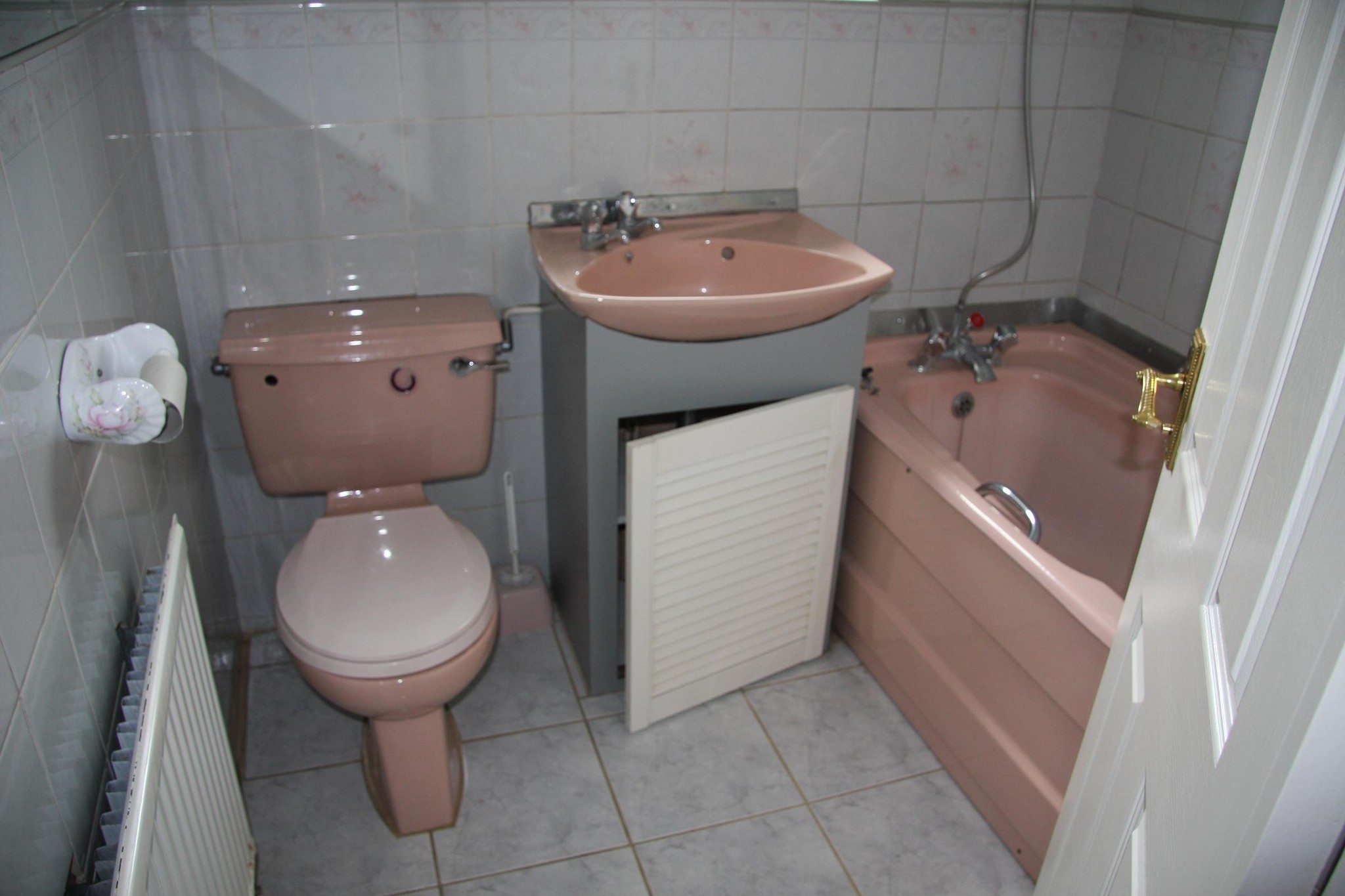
[832,322,1190,885]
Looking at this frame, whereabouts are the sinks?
[531,210,893,346]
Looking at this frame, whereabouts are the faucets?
[909,310,1019,386]
[611,191,664,244]
[572,198,632,251]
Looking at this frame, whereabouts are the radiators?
[70,512,257,896]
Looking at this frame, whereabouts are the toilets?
[203,294,519,837]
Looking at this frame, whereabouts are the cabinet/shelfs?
[538,288,864,730]
